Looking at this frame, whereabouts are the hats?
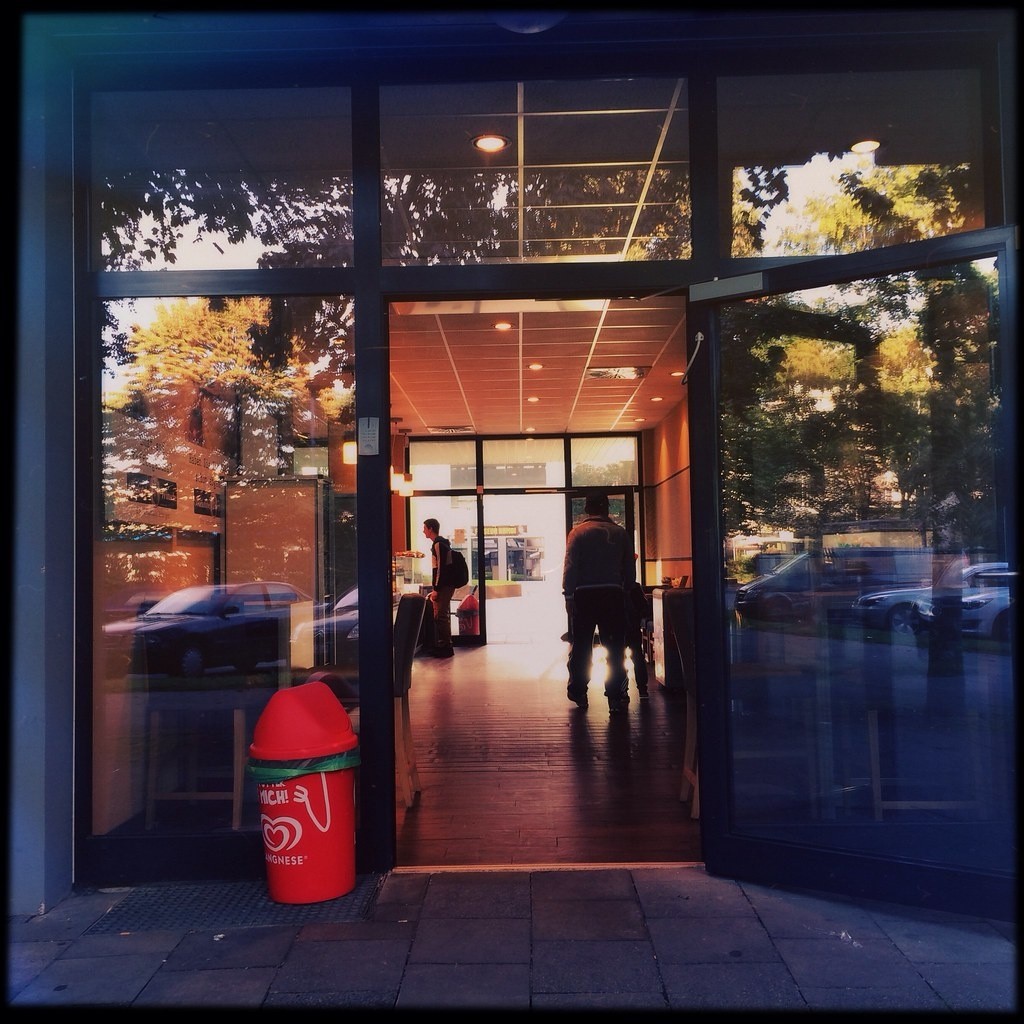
[585,490,609,510]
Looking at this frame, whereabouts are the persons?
[562,494,651,713]
[423,519,456,657]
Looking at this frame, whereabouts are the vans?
[736,544,937,623]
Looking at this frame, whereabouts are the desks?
[813,591,875,800]
[244,600,316,691]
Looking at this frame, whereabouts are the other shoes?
[608,695,630,713]
[639,686,649,698]
[568,691,589,708]
[429,646,454,658]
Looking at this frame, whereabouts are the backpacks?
[432,539,469,589]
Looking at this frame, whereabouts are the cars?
[101,582,320,681]
[852,555,1013,644]
[287,583,361,671]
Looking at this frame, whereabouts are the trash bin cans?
[246,681,358,906]
[456,594,479,635]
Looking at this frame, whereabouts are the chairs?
[139,598,257,830]
[860,588,995,823]
[665,588,822,819]
[285,592,426,806]
[640,575,690,667]
